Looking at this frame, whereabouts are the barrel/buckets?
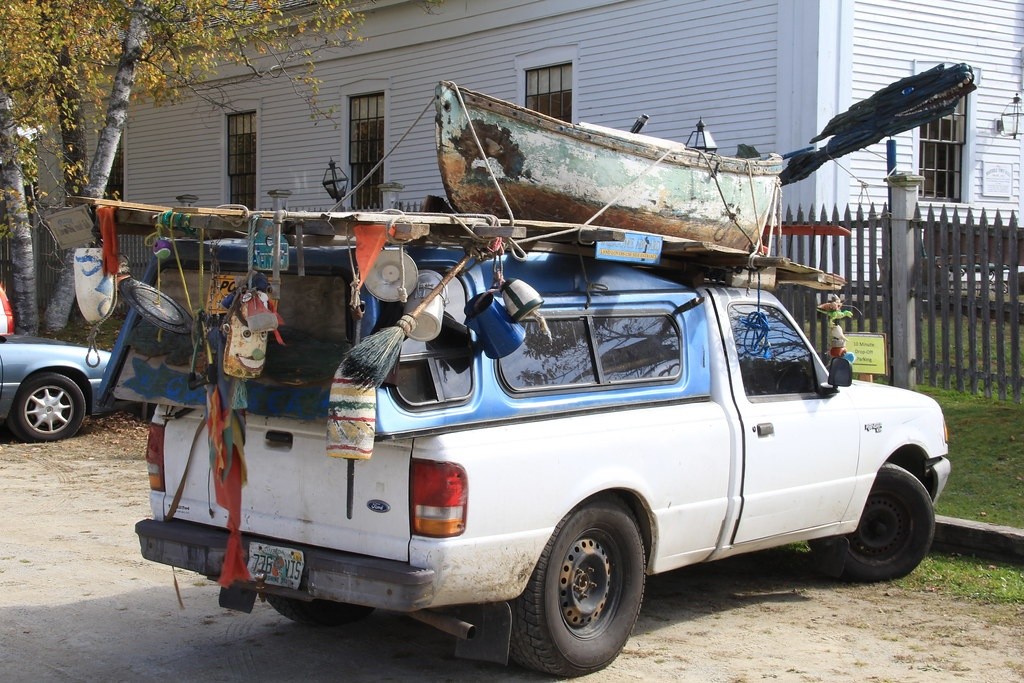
[404,269,450,342]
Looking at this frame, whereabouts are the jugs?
[464,294,526,359]
[499,277,544,322]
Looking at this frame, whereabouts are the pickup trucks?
[133,245,953,679]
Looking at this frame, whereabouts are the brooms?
[339,252,471,395]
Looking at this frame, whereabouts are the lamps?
[686,115,717,155]
[322,155,349,203]
[995,92,1024,139]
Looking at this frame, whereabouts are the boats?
[432,81,785,257]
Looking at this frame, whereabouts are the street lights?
[322,159,348,210]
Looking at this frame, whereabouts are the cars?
[0,333,136,442]
